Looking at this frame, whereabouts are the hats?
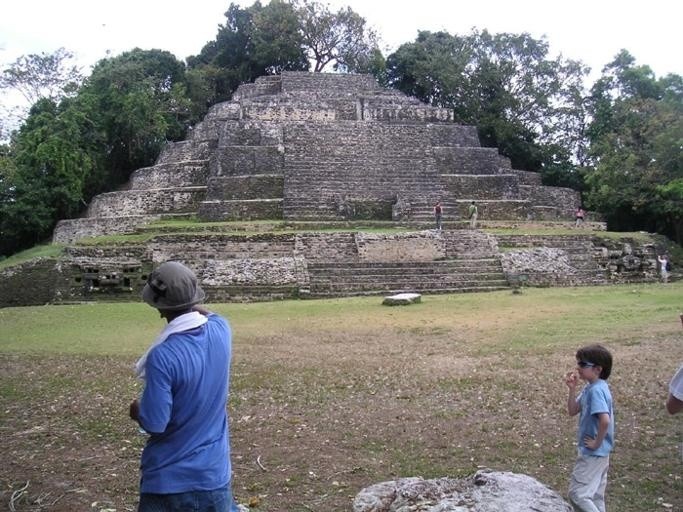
[144,261,204,312]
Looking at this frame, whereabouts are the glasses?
[578,362,595,367]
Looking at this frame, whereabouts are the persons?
[469,201,478,230]
[564,344,614,512]
[129,261,233,512]
[434,202,443,230]
[575,206,587,228]
[658,255,668,282]
[666,314,683,415]
